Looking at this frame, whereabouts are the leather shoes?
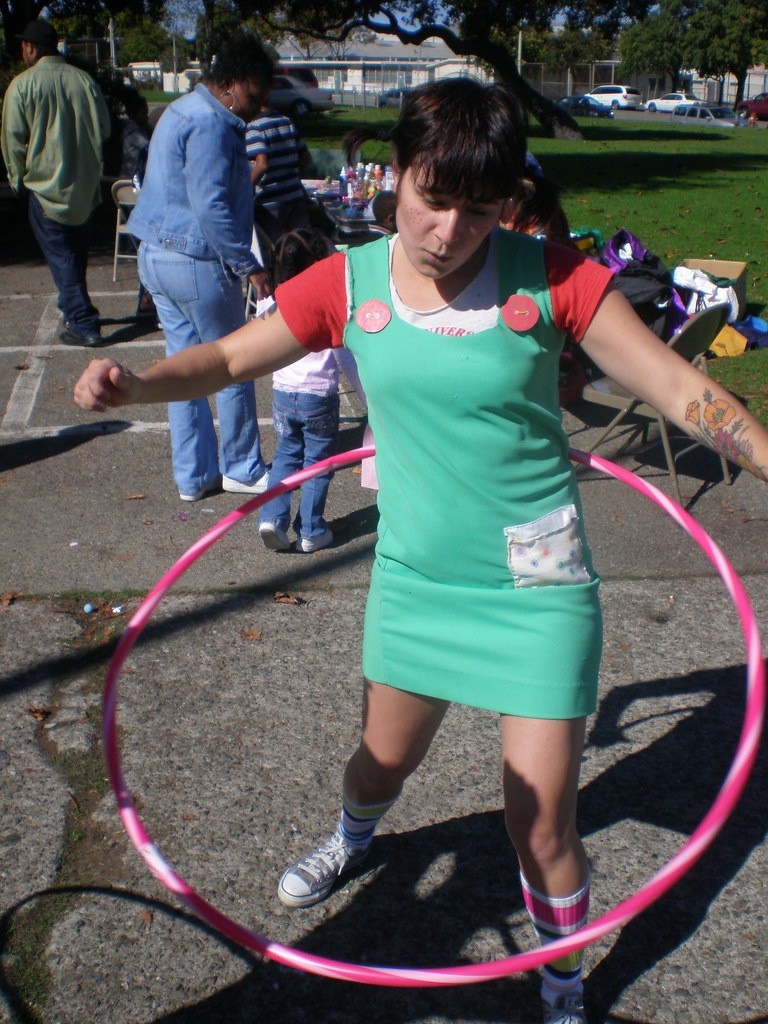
[60,331,103,346]
[63,320,69,328]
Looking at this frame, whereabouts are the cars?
[276,65,318,87]
[584,85,643,110]
[271,75,335,118]
[645,93,706,113]
[737,92,768,120]
[555,95,614,119]
[375,89,409,108]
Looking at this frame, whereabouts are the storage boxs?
[679,257,748,318]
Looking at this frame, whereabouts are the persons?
[0,19,111,346]
[118,20,572,551]
[74,78,768,1024]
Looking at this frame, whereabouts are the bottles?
[339,161,394,205]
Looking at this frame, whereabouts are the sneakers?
[296,529,333,552]
[541,982,586,1024]
[179,473,222,501]
[278,832,372,907]
[223,469,271,493]
[258,522,290,549]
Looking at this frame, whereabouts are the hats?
[14,21,58,47]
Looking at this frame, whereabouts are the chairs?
[111,179,138,282]
[576,302,732,507]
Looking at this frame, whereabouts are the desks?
[300,178,376,235]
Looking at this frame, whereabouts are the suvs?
[672,102,759,129]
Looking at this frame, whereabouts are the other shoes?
[88,242,104,256]
